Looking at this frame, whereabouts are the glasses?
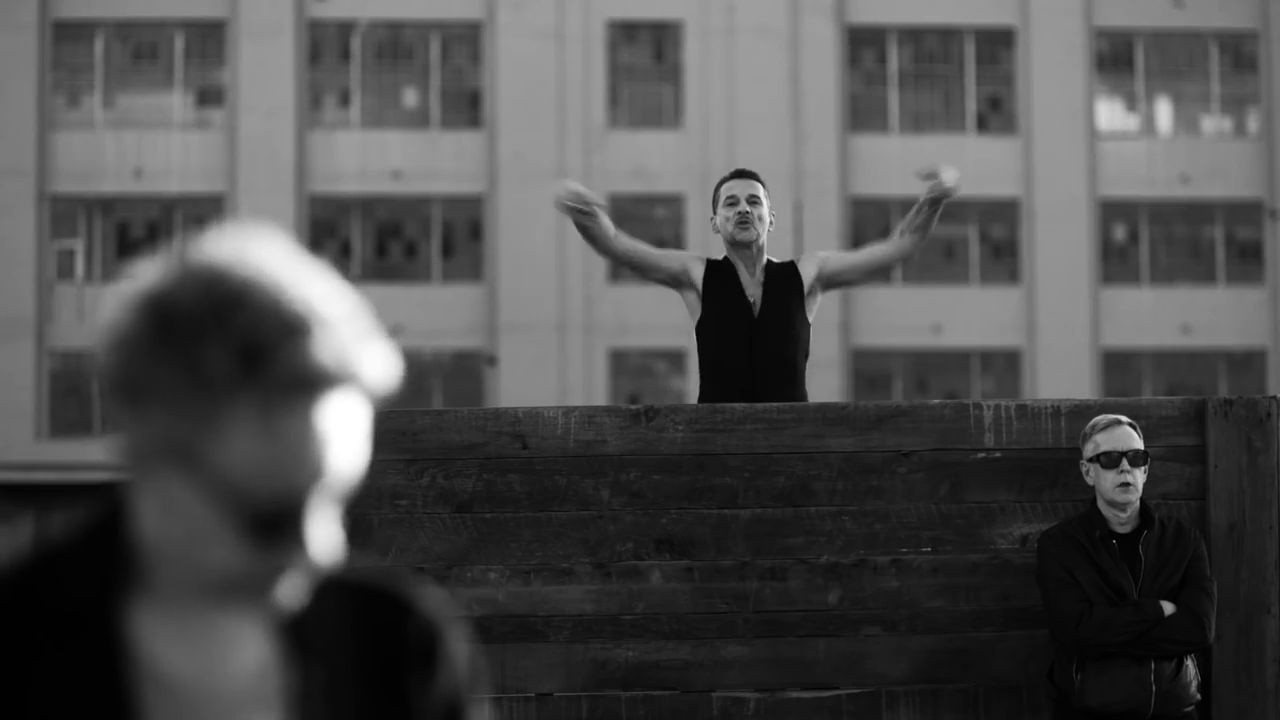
[161,439,362,563]
[1087,448,1150,470]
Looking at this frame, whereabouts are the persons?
[1036,413,1217,720]
[0,237,488,720]
[558,169,957,404]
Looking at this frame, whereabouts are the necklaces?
[748,296,755,304]
